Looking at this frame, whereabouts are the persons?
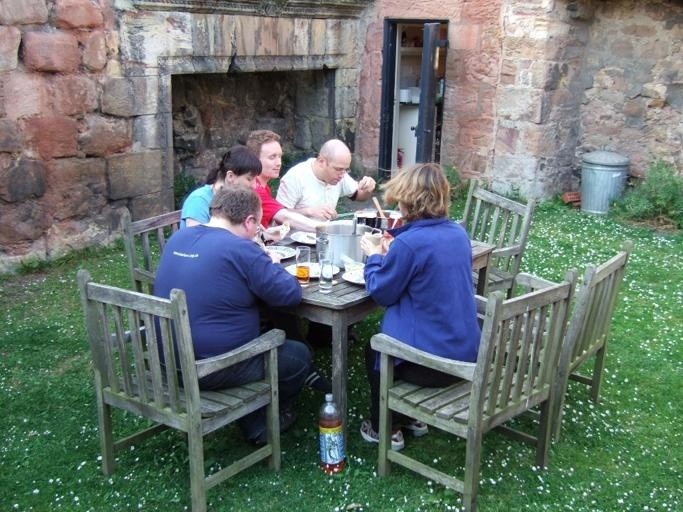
[246,129,327,396]
[151,184,312,449]
[274,136,376,222]
[179,146,262,233]
[360,161,482,452]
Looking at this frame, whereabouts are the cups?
[318,251,333,293]
[296,246,310,288]
[364,233,383,257]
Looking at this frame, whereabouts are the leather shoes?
[256,407,301,442]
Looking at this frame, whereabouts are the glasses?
[252,221,262,235]
[327,162,352,175]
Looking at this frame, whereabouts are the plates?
[259,245,298,261]
[342,271,366,285]
[291,231,317,245]
[284,262,340,277]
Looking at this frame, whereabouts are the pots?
[315,223,382,270]
[355,208,403,229]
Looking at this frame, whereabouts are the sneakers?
[357,419,405,451]
[394,416,429,438]
[302,366,332,392]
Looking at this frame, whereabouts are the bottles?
[319,393,345,473]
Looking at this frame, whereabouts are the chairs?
[120,209,184,295]
[471,239,633,441]
[77,268,285,512]
[369,268,578,510]
[453,176,536,299]
